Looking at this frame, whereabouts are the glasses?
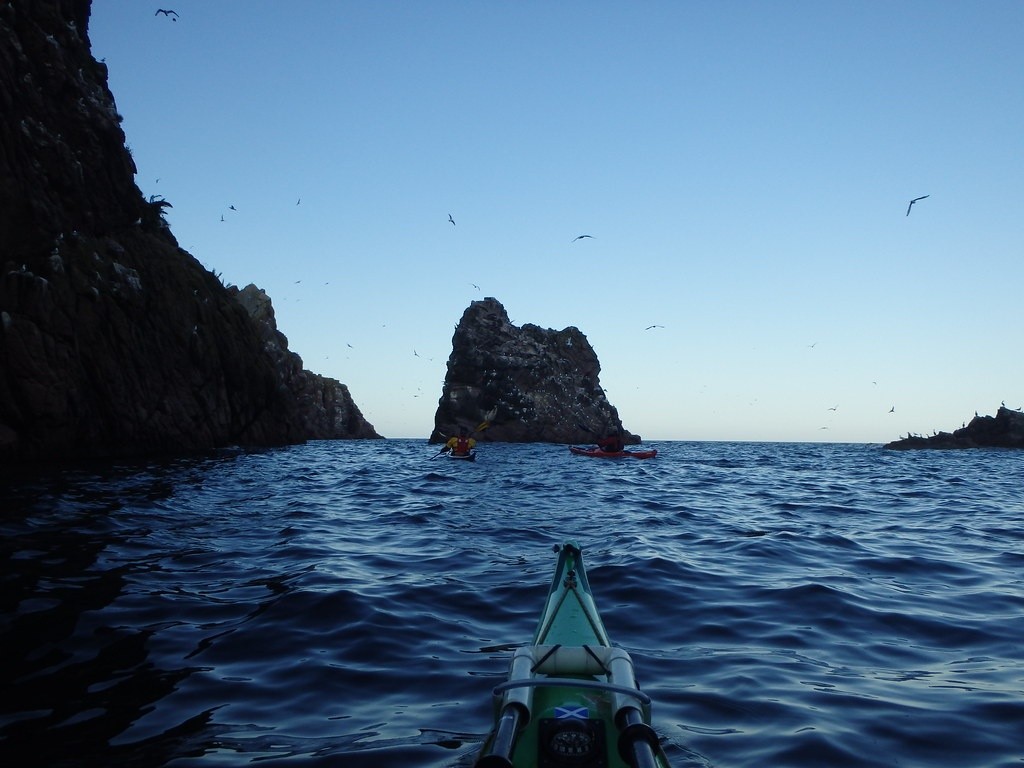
[460,432,467,435]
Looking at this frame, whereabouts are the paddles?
[578,423,606,440]
[429,420,490,462]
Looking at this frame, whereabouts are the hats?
[612,426,617,431]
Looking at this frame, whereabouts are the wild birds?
[906,195,930,217]
[154,177,895,430]
[155,9,180,17]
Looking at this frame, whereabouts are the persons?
[597,430,624,452]
[440,426,477,456]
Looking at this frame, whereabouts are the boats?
[446,450,475,462]
[569,446,657,459]
